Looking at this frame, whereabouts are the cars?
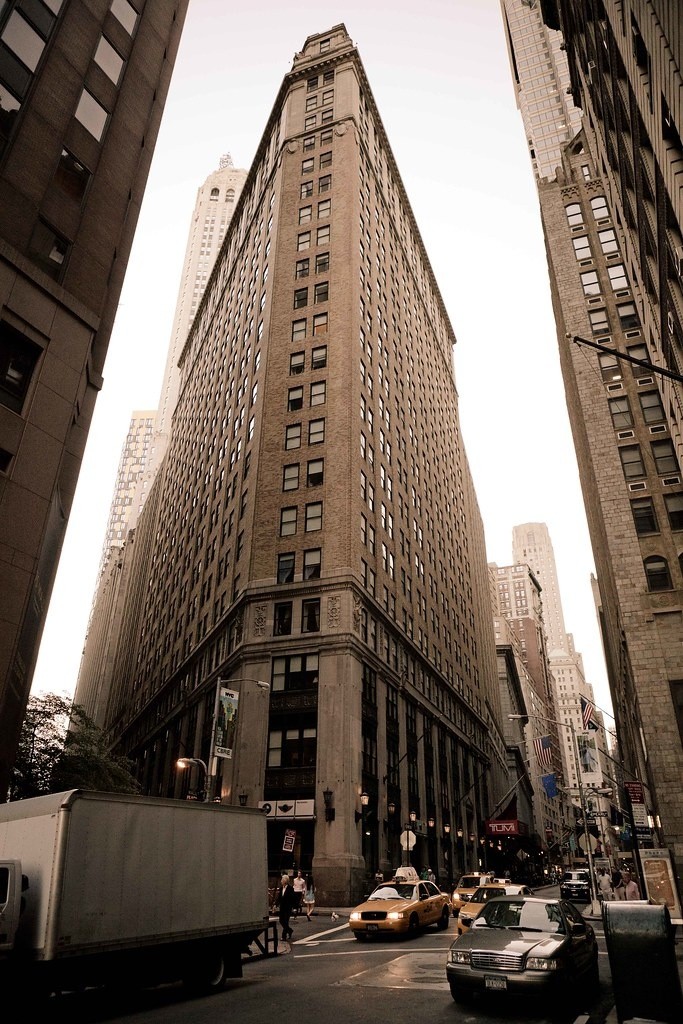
[445,895,599,1013]
[456,879,536,939]
[349,867,452,941]
[451,872,496,918]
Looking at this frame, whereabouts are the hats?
[428,869,432,871]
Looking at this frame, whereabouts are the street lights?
[177,674,273,801]
[505,713,613,914]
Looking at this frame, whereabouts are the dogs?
[331,912,339,922]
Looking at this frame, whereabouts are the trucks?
[0,788,281,1017]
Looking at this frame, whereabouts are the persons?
[420,867,436,884]
[375,870,383,881]
[552,871,562,884]
[293,869,306,918]
[273,875,295,941]
[595,866,640,900]
[302,875,316,921]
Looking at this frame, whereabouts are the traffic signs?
[587,810,610,818]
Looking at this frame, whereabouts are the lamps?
[426,818,435,828]
[444,824,450,833]
[355,791,370,824]
[404,811,417,830]
[470,832,476,841]
[457,828,464,837]
[213,797,222,803]
[239,790,248,807]
[384,803,395,829]
[480,837,485,845]
[323,787,335,822]
[490,841,494,848]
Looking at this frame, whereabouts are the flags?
[588,719,599,732]
[581,696,594,730]
[610,805,623,826]
[542,774,558,798]
[533,735,553,766]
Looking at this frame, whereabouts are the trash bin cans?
[601,898,683,1024]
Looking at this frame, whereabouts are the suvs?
[559,869,591,902]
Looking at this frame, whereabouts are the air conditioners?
[588,61,598,84]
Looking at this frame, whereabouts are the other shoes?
[287,928,293,940]
[280,935,286,941]
[306,915,311,921]
